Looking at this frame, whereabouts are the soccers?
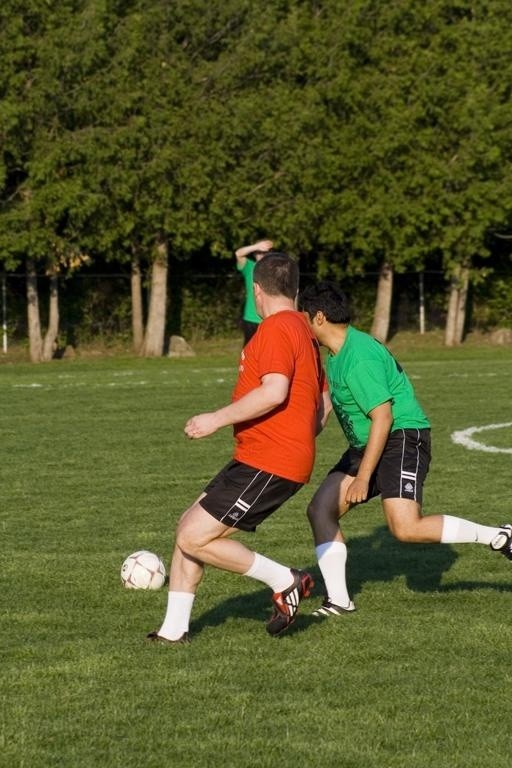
[121,550,166,590]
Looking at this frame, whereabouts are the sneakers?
[146,628,192,649]
[263,564,315,638]
[488,522,512,561]
[307,596,360,618]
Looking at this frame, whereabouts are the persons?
[232,238,273,343]
[300,281,511,617]
[146,255,334,643]
